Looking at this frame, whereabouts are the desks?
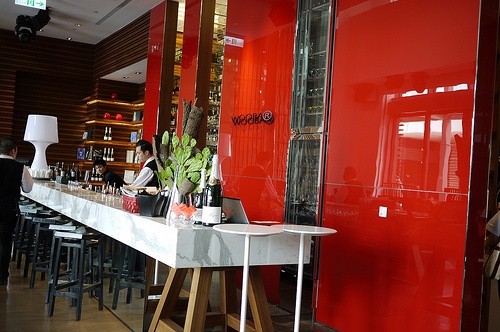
[271,224,338,332]
[213,224,283,332]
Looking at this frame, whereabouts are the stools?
[10,197,145,322]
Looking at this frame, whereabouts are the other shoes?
[0,278,8,285]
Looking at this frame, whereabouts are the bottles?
[202,155,223,226]
[103,148,107,161]
[104,127,108,140]
[86,182,124,196]
[207,79,221,154]
[108,127,112,140]
[111,148,114,161]
[170,84,179,136]
[54,161,103,182]
[107,148,111,161]
[193,168,208,226]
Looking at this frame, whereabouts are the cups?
[26,166,54,178]
[67,180,82,191]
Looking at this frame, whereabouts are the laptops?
[222,197,280,224]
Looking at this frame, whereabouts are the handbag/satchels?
[483,242,500,281]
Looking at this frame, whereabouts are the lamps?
[14,24,37,44]
[22,114,59,171]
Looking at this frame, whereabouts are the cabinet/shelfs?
[84,100,144,167]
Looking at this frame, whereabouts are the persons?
[331,166,367,203]
[116,139,160,290]
[238,151,281,221]
[93,159,128,195]
[485,193,500,251]
[0,140,33,285]
